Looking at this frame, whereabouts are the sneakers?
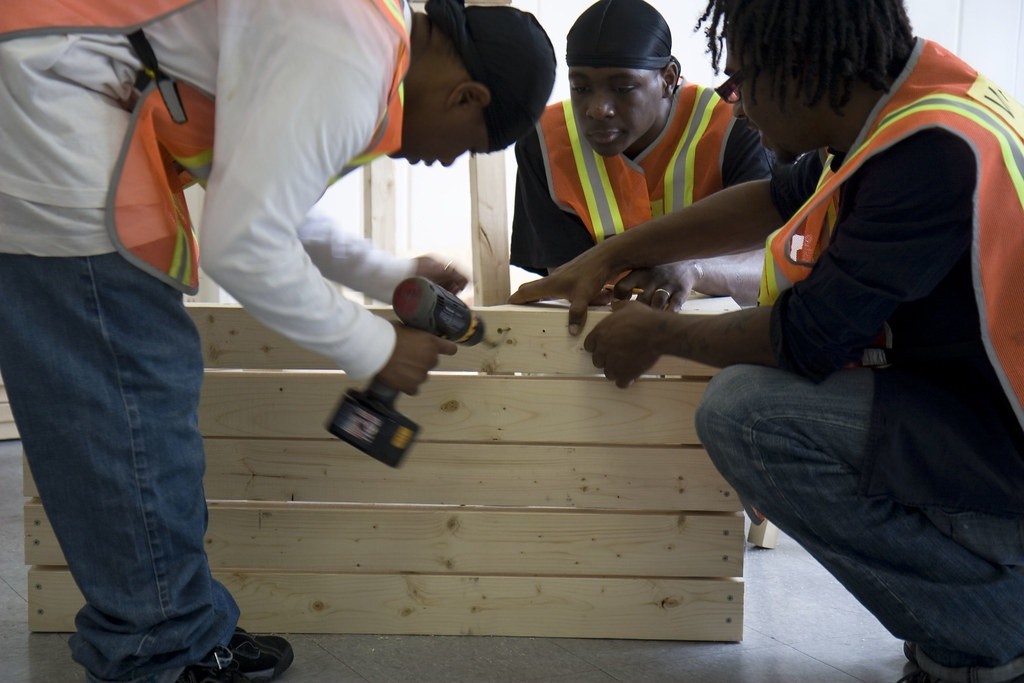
[175,627,292,683]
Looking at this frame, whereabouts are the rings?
[656,289,670,297]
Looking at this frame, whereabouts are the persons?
[506,0,1024,683]
[0,0,557,683]
[509,0,788,314]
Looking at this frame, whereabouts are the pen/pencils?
[602,284,645,294]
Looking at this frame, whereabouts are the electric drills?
[325,275,497,468]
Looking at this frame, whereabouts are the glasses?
[717,64,759,102]
